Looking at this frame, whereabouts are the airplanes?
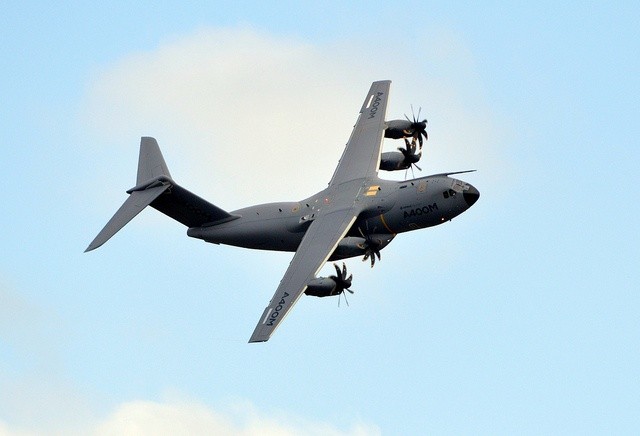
[82,81,480,344]
